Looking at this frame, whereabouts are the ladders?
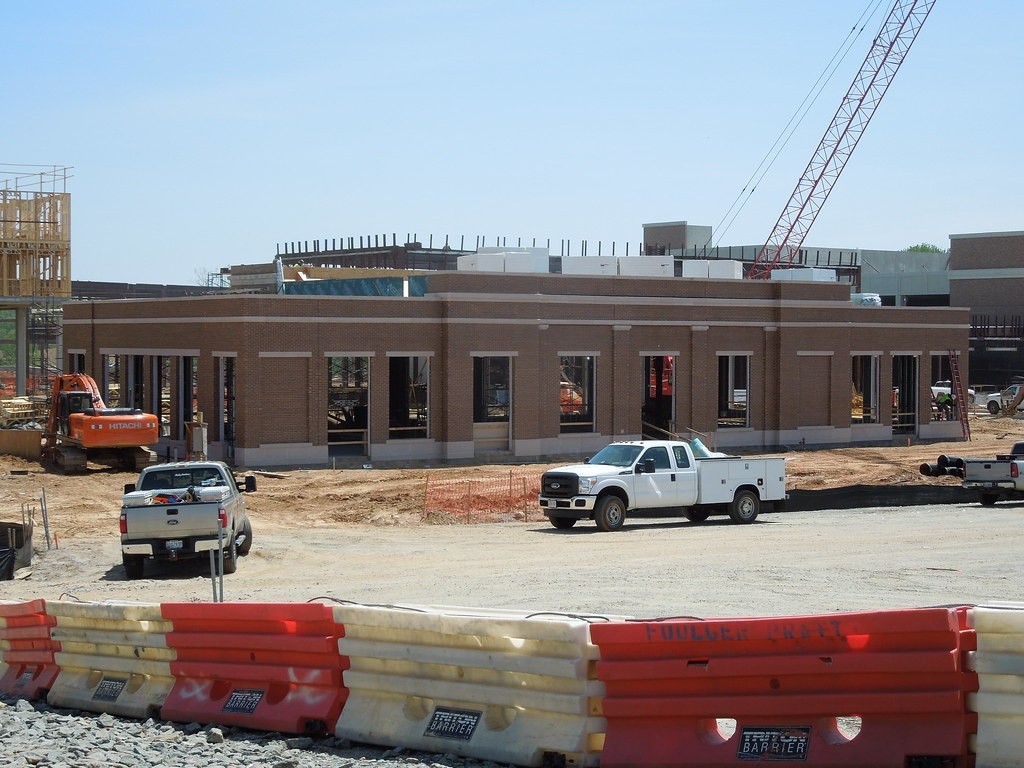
[948,348,973,441]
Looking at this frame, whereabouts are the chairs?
[632,451,646,462]
[159,479,176,489]
[655,451,668,467]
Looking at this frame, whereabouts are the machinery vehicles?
[647,0,939,395]
[42,372,158,476]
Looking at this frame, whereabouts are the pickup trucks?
[984,384,1024,415]
[961,441,1024,507]
[118,460,259,574]
[931,380,976,405]
[537,439,790,532]
